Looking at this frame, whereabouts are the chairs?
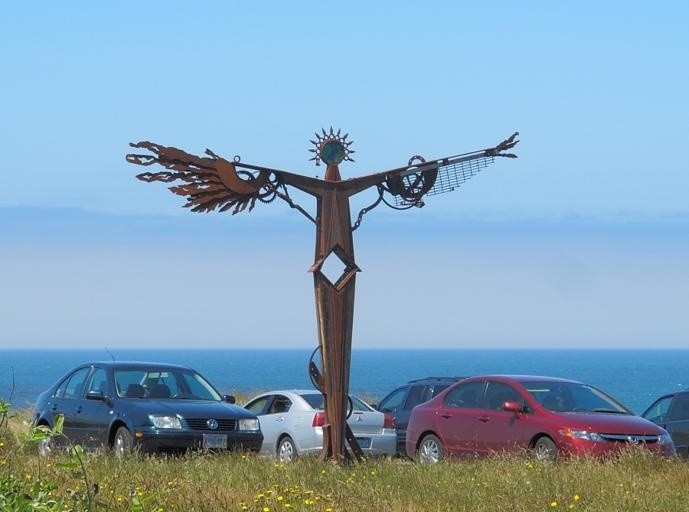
[150,384,172,399]
[126,384,145,396]
[271,401,286,411]
[542,397,567,410]
[461,391,477,407]
[75,384,83,396]
[98,382,121,396]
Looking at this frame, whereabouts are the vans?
[369,376,469,462]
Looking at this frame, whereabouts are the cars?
[639,391,689,460]
[32,345,265,464]
[241,388,397,465]
[405,374,678,466]
